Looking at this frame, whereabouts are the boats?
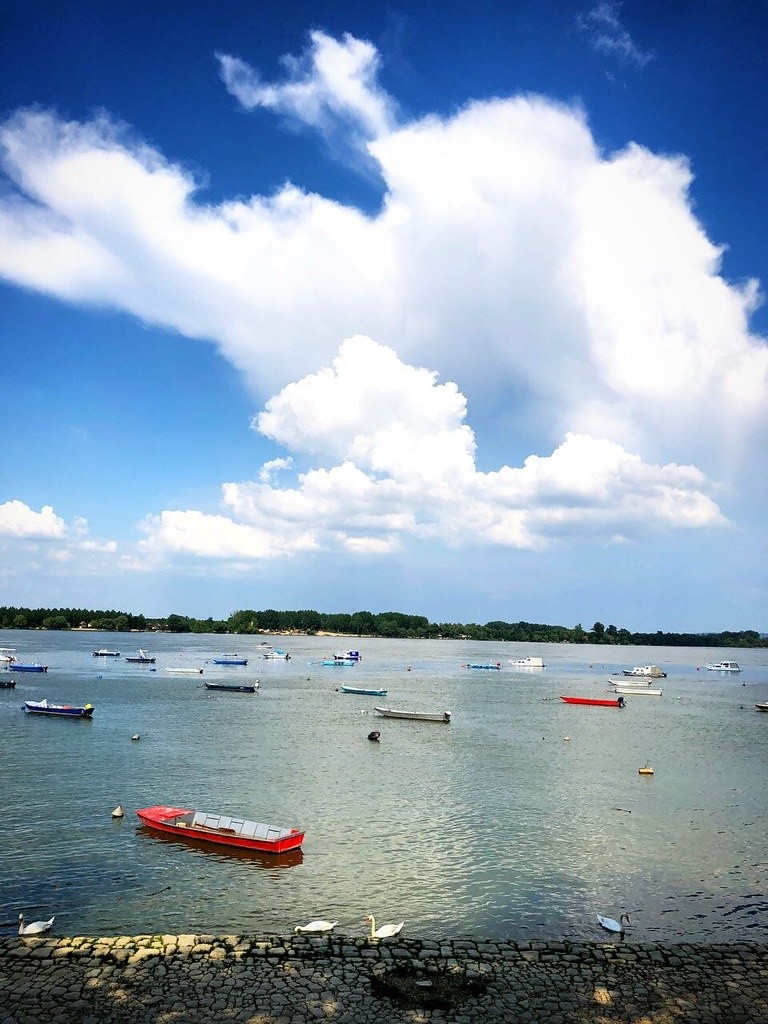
[0,648,17,662]
[8,661,49,673]
[560,696,627,708]
[205,679,261,693]
[622,662,669,678]
[135,806,307,854]
[507,655,547,667]
[340,685,388,696]
[466,658,502,671]
[607,677,653,687]
[256,641,274,648]
[92,648,121,656]
[319,648,363,666]
[0,679,17,689]
[754,702,768,712]
[124,648,156,663]
[20,698,95,717]
[374,707,452,725]
[703,660,743,672]
[613,688,663,697]
[262,648,292,660]
[212,652,249,666]
[165,667,204,674]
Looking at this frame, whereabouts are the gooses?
[596,912,630,933]
[294,921,338,932]
[17,914,55,934]
[366,915,404,938]
